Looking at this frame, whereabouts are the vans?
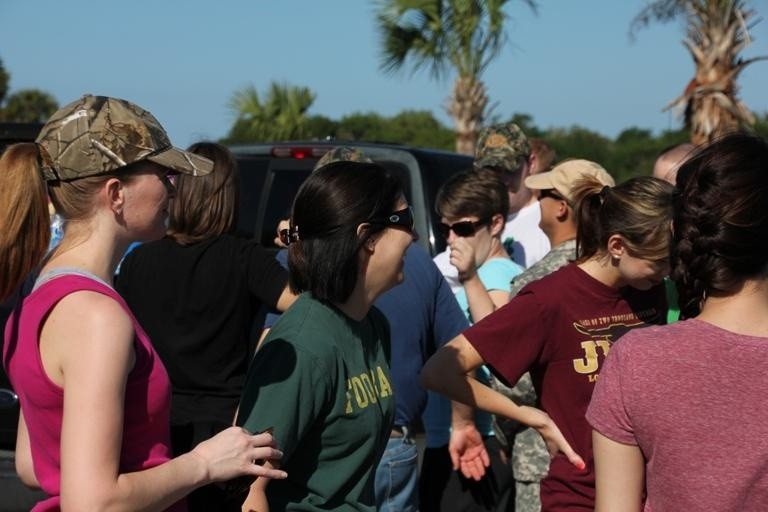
[222,141,483,260]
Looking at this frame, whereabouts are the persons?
[118,142,307,512]
[232,143,491,510]
[0,94,287,511]
[430,121,768,512]
[222,161,421,511]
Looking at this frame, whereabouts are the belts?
[388,426,417,439]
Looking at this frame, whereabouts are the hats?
[472,120,533,173]
[311,145,373,173]
[32,93,216,183]
[523,158,617,205]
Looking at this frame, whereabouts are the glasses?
[370,204,416,234]
[432,218,487,238]
[537,189,564,200]
